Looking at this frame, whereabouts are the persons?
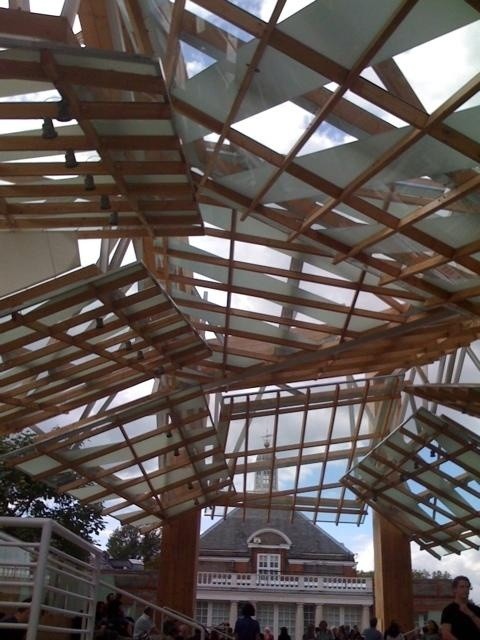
[1,595,48,640]
[439,577,480,639]
